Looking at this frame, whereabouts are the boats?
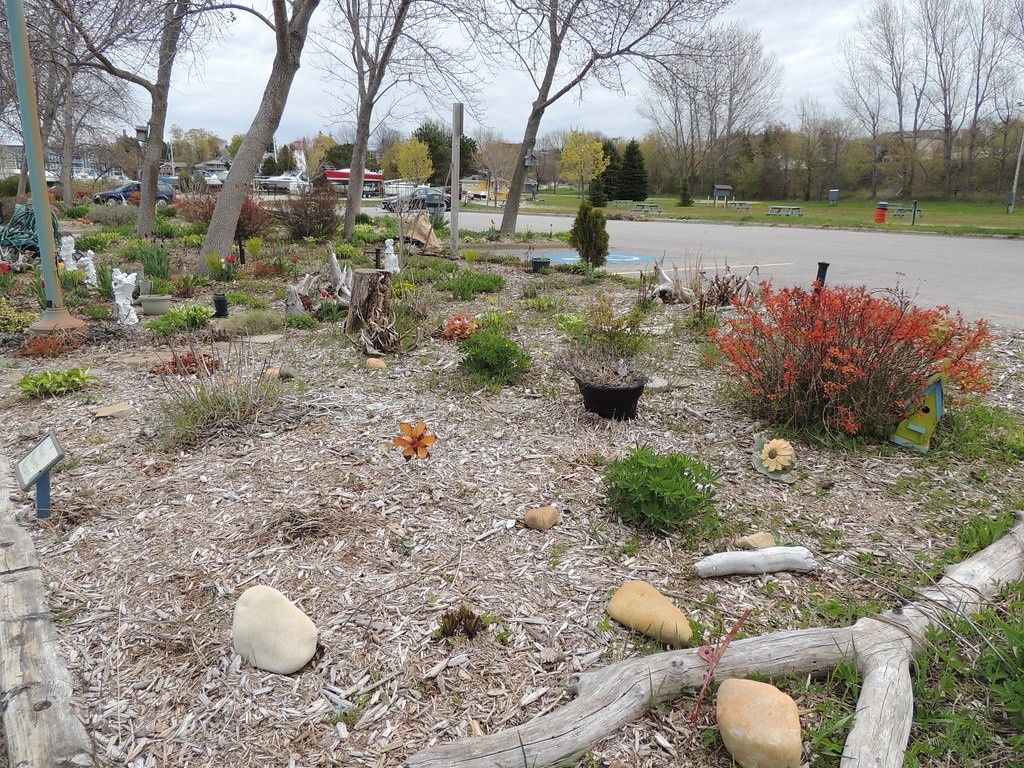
[256,168,313,191]
[160,138,222,188]
[13,167,126,185]
[326,165,384,184]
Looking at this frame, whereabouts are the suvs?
[92,182,174,207]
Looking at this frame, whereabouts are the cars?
[383,187,451,213]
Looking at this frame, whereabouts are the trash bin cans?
[548,182,554,191]
[829,190,840,201]
[874,202,888,223]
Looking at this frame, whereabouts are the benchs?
[612,204,633,208]
[725,205,750,210]
[765,211,806,217]
[891,213,925,217]
[630,208,664,214]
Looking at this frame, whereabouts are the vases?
[533,257,551,271]
[137,294,172,315]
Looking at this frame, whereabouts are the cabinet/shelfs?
[499,203,520,209]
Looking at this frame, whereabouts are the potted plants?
[560,307,649,423]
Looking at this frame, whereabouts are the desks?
[895,208,925,223]
[613,200,633,207]
[728,202,748,209]
[501,200,520,208]
[635,204,661,213]
[768,206,802,216]
